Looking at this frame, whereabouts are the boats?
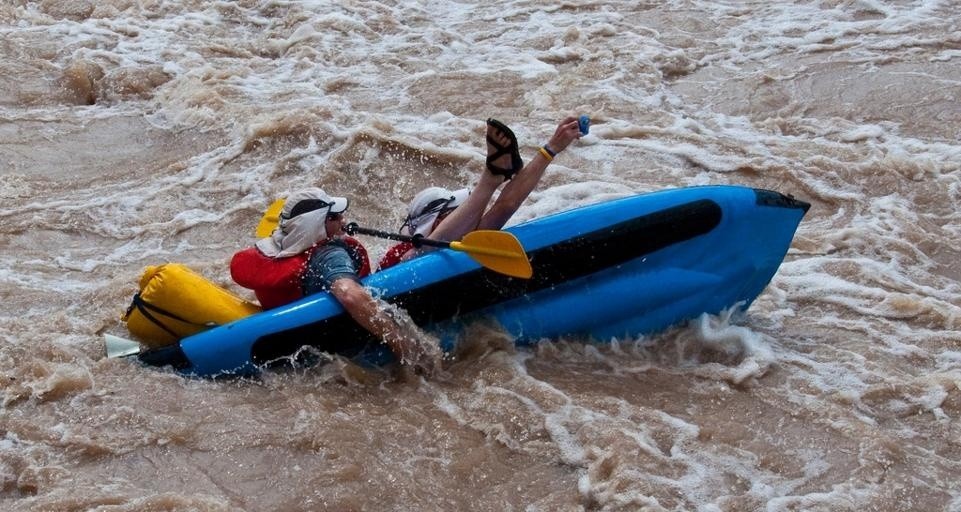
[135,183,815,383]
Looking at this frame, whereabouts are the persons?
[230,117,523,372]
[380,116,584,270]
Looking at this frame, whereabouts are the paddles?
[257,199,534,280]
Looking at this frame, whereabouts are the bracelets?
[540,148,553,161]
[545,144,556,156]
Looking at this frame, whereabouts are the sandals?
[486,119,522,179]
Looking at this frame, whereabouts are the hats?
[407,187,473,217]
[280,186,348,217]
